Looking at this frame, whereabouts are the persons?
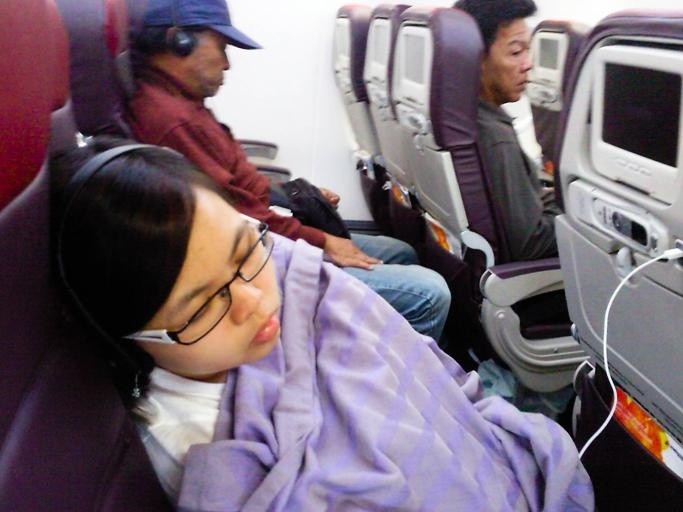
[47,135,608,512]
[125,0,452,346]
[450,0,565,261]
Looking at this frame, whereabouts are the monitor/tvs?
[332,21,352,72]
[592,47,683,205]
[533,32,570,84]
[396,27,432,103]
[368,23,392,84]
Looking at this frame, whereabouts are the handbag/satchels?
[279,179,350,239]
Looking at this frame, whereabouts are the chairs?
[1,3,305,512]
[546,3,682,512]
[325,4,587,415]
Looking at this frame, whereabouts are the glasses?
[126,215,275,346]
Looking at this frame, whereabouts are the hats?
[129,0,262,51]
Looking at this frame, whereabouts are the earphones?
[172,31,195,56]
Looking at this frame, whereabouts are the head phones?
[71,143,216,401]
